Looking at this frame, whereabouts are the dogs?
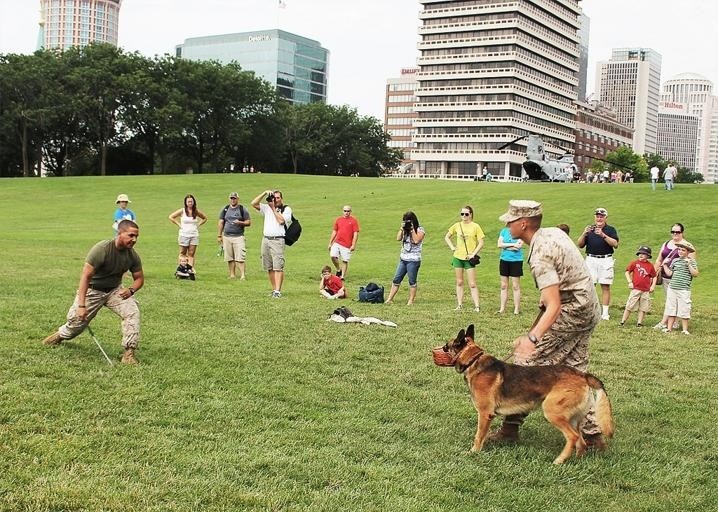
[431,324,615,465]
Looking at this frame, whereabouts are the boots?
[42,331,64,345]
[484,424,518,443]
[582,434,607,450]
[120,348,139,365]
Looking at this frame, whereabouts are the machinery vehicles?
[499,135,580,183]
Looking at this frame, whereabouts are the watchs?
[527,331,539,345]
[128,286,135,295]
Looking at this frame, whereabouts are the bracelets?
[78,303,87,310]
[602,233,608,239]
[217,235,222,239]
[409,230,414,233]
[400,227,403,232]
[627,280,632,284]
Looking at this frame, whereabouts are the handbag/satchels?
[284,220,301,246]
[469,255,479,266]
[655,268,661,285]
[358,286,383,304]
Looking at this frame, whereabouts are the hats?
[594,208,606,214]
[674,242,695,252]
[228,192,238,198]
[498,199,541,222]
[635,246,651,259]
[115,194,131,203]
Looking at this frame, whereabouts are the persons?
[318,266,350,302]
[249,190,293,300]
[173,255,197,283]
[659,240,698,336]
[619,246,659,327]
[579,207,618,321]
[383,212,427,307]
[496,221,523,316]
[653,222,696,331]
[554,224,570,235]
[326,204,359,281]
[223,156,256,173]
[168,195,207,268]
[565,162,679,191]
[112,194,137,237]
[485,172,492,182]
[486,198,608,451]
[41,219,144,366]
[483,166,488,179]
[444,206,486,313]
[217,192,253,282]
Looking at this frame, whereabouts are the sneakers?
[269,290,280,298]
[229,275,246,280]
[385,299,414,305]
[336,270,344,280]
[496,309,520,314]
[600,313,692,335]
[455,305,480,312]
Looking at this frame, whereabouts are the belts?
[586,254,611,258]
[264,236,284,239]
[90,286,113,293]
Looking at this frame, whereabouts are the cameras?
[470,255,480,266]
[266,194,276,203]
[590,225,597,230]
[403,220,412,236]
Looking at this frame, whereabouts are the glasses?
[460,213,468,216]
[670,231,681,234]
[344,210,350,212]
[594,210,605,212]
